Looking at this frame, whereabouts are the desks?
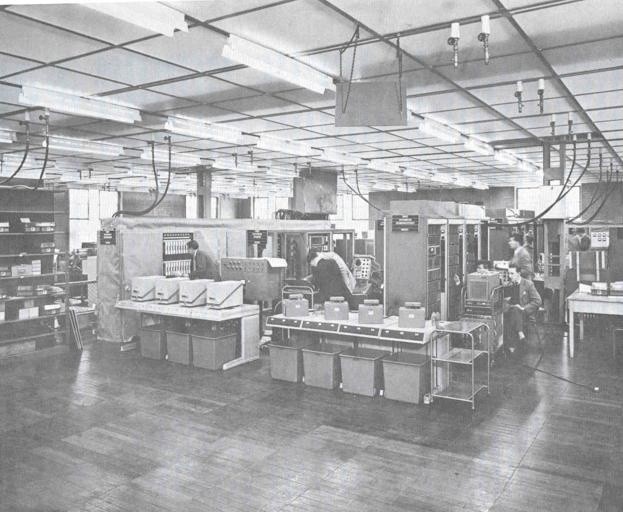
[565,288,623,358]
[114,299,260,371]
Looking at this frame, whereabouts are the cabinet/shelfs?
[0,209,69,344]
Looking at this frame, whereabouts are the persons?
[305,247,358,294]
[186,240,221,281]
[503,263,543,354]
[306,252,352,303]
[577,228,590,250]
[507,233,532,282]
[568,228,579,251]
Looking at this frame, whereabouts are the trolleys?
[458,286,505,368]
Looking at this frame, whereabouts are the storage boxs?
[140,322,435,406]
